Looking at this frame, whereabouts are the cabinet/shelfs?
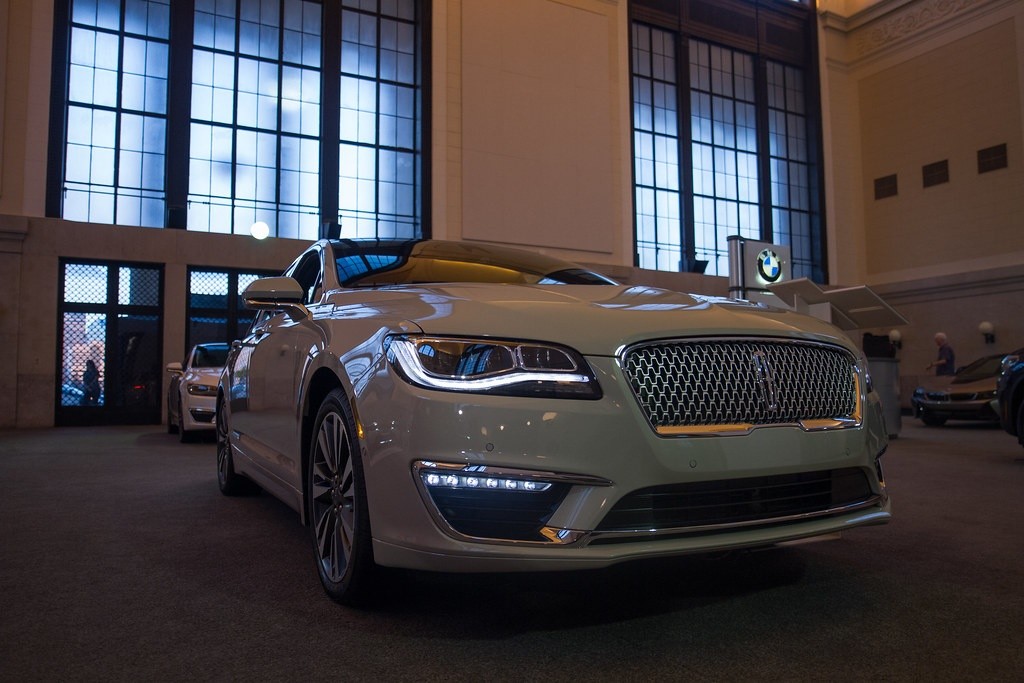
[867,358,901,439]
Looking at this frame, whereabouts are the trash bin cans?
[867,357,902,439]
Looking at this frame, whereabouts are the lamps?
[889,330,900,349]
[979,322,994,345]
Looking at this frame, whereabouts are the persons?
[81,358,102,405]
[926,328,955,377]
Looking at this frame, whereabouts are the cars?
[210,234,899,606]
[910,352,1011,427]
[163,341,241,443]
